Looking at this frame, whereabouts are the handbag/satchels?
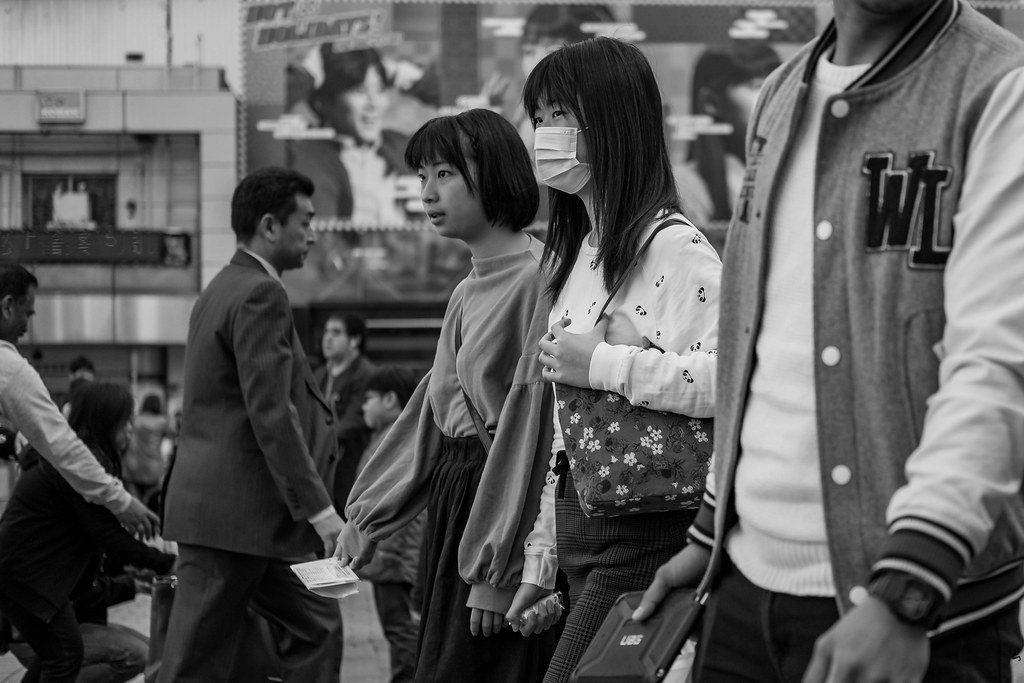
[143,575,281,683]
[555,218,736,518]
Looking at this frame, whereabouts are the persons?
[504,30,725,683]
[510,3,632,222]
[280,30,509,250]
[631,0,1024,683]
[155,163,343,683]
[0,259,176,683]
[332,104,563,683]
[307,309,429,683]
[672,35,787,222]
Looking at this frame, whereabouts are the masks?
[534,127,592,194]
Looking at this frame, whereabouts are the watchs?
[869,568,946,633]
